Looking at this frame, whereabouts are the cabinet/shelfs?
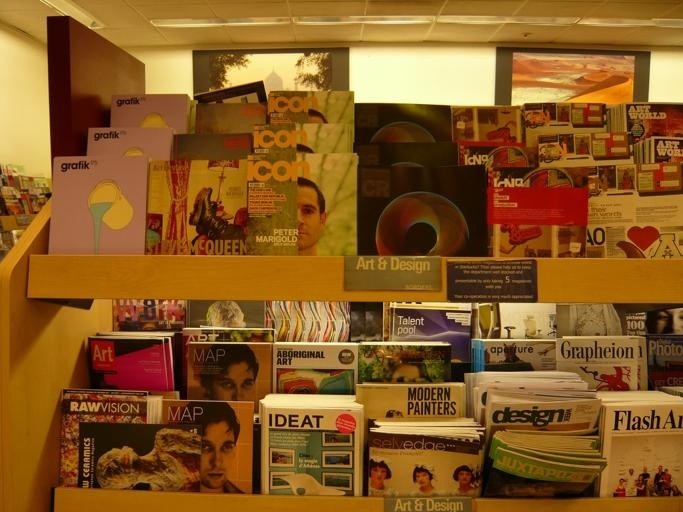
[22,248,683,510]
